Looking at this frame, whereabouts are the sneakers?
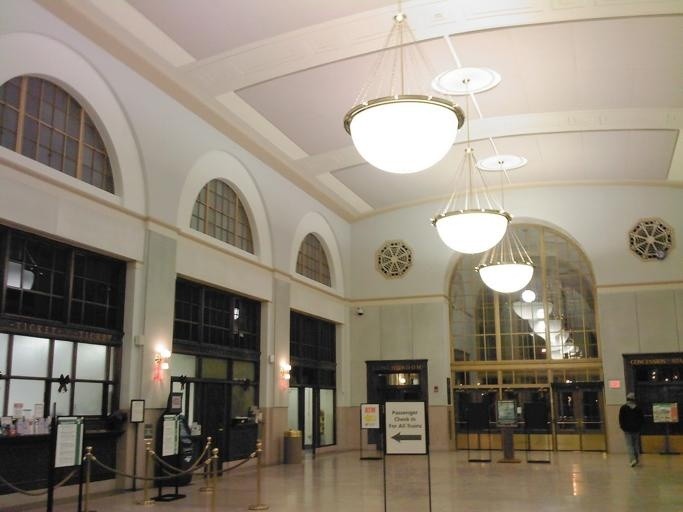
[629,458,640,467]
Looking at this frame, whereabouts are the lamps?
[430,68,511,253]
[152,349,172,383]
[512,300,580,359]
[280,363,291,384]
[345,2,464,176]
[474,154,537,295]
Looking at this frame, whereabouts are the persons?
[618,392,642,468]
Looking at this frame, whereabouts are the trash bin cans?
[284,429,303,464]
